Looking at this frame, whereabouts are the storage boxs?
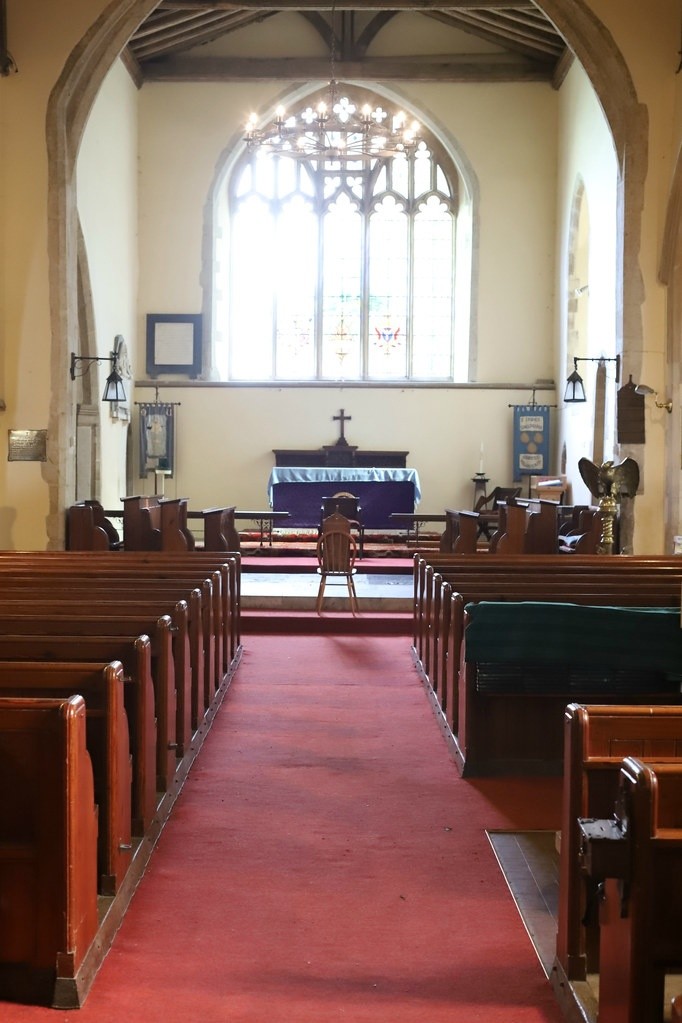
[530,474,567,503]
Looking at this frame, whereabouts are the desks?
[268,466,423,534]
[388,512,501,548]
[104,508,291,546]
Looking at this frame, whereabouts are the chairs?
[315,496,366,619]
[473,487,523,543]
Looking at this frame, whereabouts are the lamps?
[68,350,126,402]
[241,0,427,166]
[562,355,620,402]
[635,385,673,413]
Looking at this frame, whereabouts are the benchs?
[0,550,244,1011]
[411,553,682,1023]
